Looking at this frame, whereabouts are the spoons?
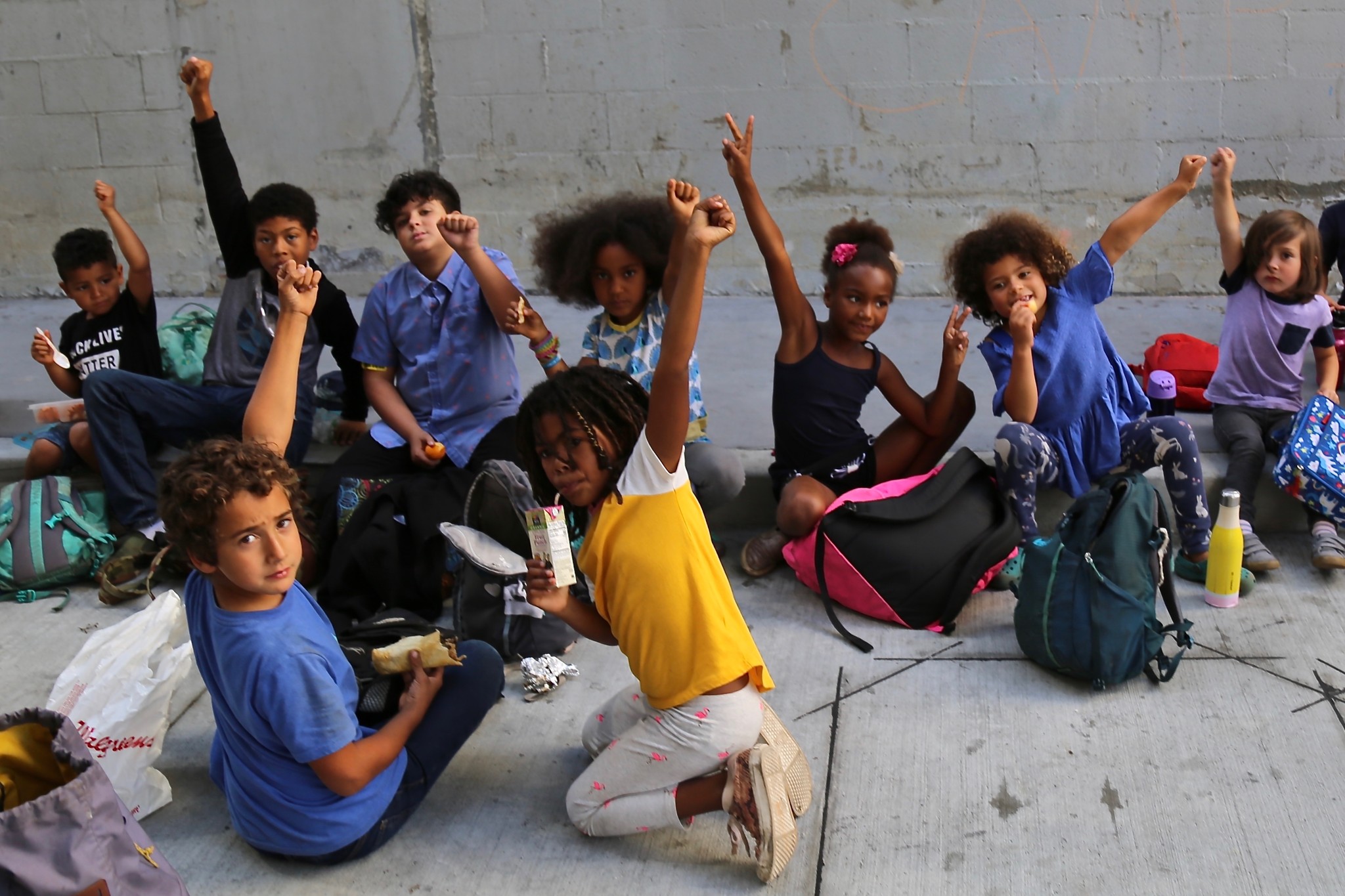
[36,327,70,368]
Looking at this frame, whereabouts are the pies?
[517,296,525,323]
[372,629,467,674]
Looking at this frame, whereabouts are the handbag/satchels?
[156,300,219,387]
[48,586,209,820]
[0,708,191,896]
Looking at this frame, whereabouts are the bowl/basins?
[27,398,87,424]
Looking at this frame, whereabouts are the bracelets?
[529,331,562,370]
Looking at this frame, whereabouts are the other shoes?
[97,530,175,607]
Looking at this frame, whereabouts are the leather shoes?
[739,526,792,576]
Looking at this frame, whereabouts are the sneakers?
[720,744,799,885]
[759,698,813,815]
[1241,534,1280,570]
[1312,532,1345,568]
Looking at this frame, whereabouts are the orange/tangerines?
[425,442,446,459]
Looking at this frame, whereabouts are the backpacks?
[1128,331,1219,411]
[780,445,1025,653]
[1267,394,1345,530]
[1013,468,1194,693]
[0,475,116,611]
[454,459,584,662]
[317,480,444,626]
[338,608,448,720]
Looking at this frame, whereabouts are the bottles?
[1145,370,1177,418]
[1205,487,1243,608]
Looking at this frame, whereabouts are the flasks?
[1316,310,1345,392]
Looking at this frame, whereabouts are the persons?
[156,260,505,867]
[514,196,813,885]
[721,110,976,579]
[1211,147,1345,572]
[84,57,372,607]
[508,178,746,507]
[23,180,165,479]
[308,167,531,580]
[942,154,1257,600]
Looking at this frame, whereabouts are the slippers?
[990,547,1025,591]
[1173,548,1255,596]
